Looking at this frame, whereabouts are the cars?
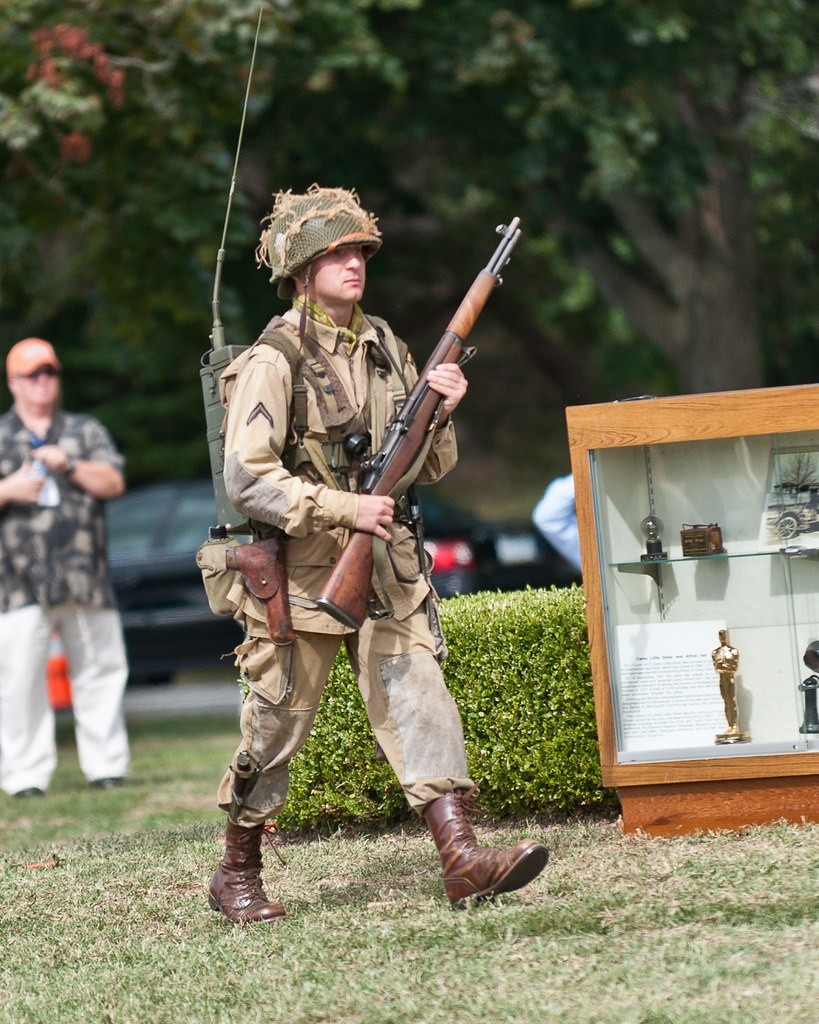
[94,477,585,686]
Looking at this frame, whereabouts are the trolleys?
[766,511,812,541]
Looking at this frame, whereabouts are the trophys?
[712,629,752,746]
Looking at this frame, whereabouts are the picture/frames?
[755,444,819,545]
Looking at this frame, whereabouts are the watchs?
[60,454,77,478]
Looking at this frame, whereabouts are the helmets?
[265,189,385,298]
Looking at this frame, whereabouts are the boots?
[206,817,288,925]
[424,792,552,912]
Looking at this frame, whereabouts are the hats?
[6,337,60,377]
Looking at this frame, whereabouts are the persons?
[532,474,582,574]
[0,338,134,797]
[194,182,552,928]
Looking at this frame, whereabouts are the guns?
[316,216,523,630]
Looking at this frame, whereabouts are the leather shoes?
[93,778,122,791]
[15,787,44,799]
